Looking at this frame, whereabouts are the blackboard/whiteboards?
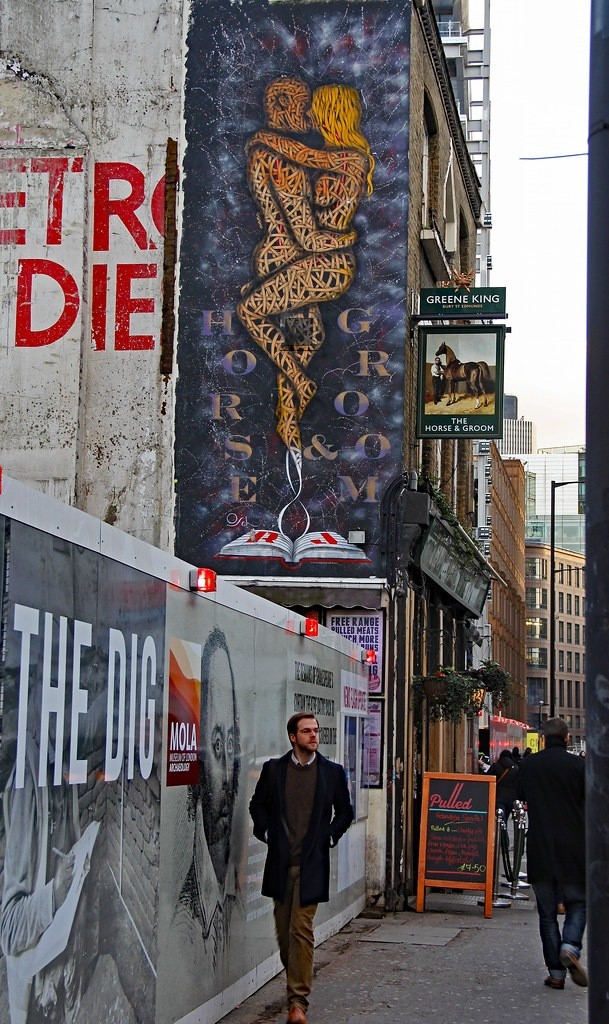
[419,771,497,889]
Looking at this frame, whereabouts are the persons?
[430,356,445,405]
[0,634,109,1024]
[579,750,586,761]
[486,749,520,847]
[523,747,532,758]
[165,623,246,970]
[247,712,353,1024]
[504,717,589,991]
[510,747,521,763]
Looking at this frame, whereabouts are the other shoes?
[558,903,565,914]
[544,976,565,990]
[560,951,588,988]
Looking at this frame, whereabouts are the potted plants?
[466,659,513,709]
[412,666,480,726]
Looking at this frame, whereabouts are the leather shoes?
[287,1006,307,1024]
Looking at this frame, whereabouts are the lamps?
[348,529,366,546]
[456,617,483,648]
